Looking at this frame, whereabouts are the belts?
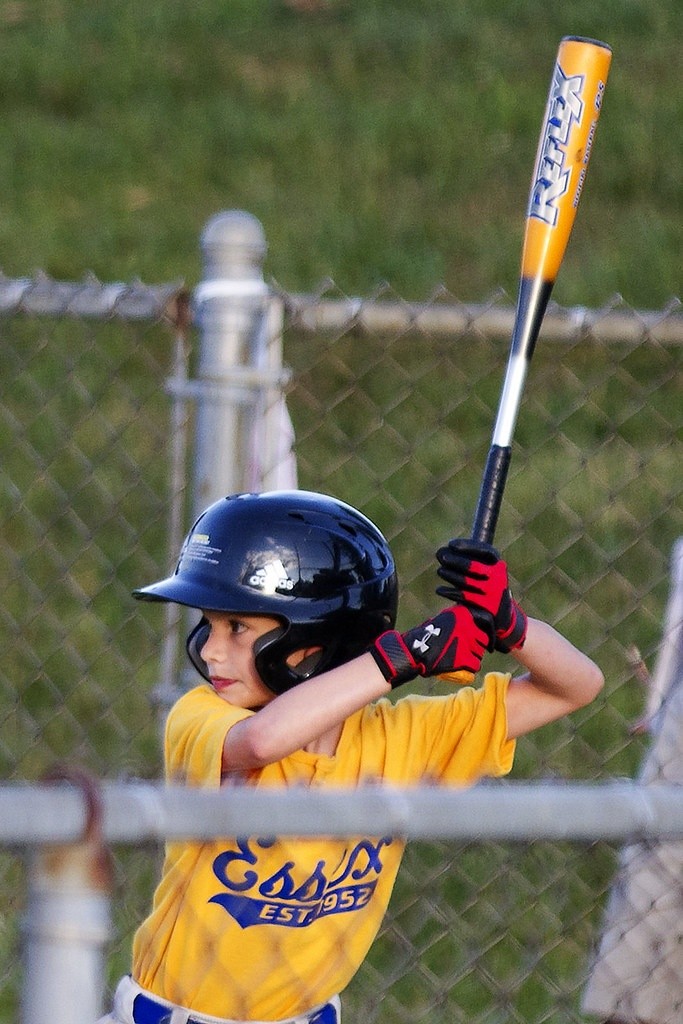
[133,993,337,1024]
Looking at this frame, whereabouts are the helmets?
[127,488,398,696]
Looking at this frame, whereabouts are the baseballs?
[434,35,616,688]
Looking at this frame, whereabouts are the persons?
[95,490,605,1024]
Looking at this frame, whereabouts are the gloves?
[364,605,499,689]
[437,538,528,654]
